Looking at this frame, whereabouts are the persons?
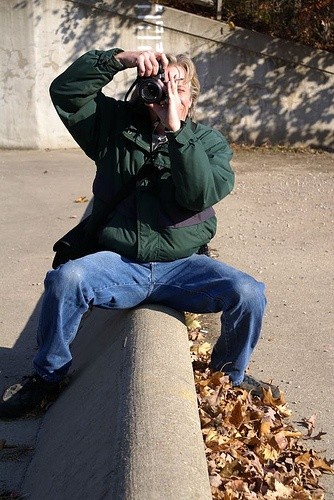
[2,49,279,422]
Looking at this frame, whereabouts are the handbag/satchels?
[52,214,92,270]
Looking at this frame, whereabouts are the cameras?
[137,62,168,103]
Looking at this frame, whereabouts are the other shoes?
[0,372,65,420]
[237,373,280,401]
